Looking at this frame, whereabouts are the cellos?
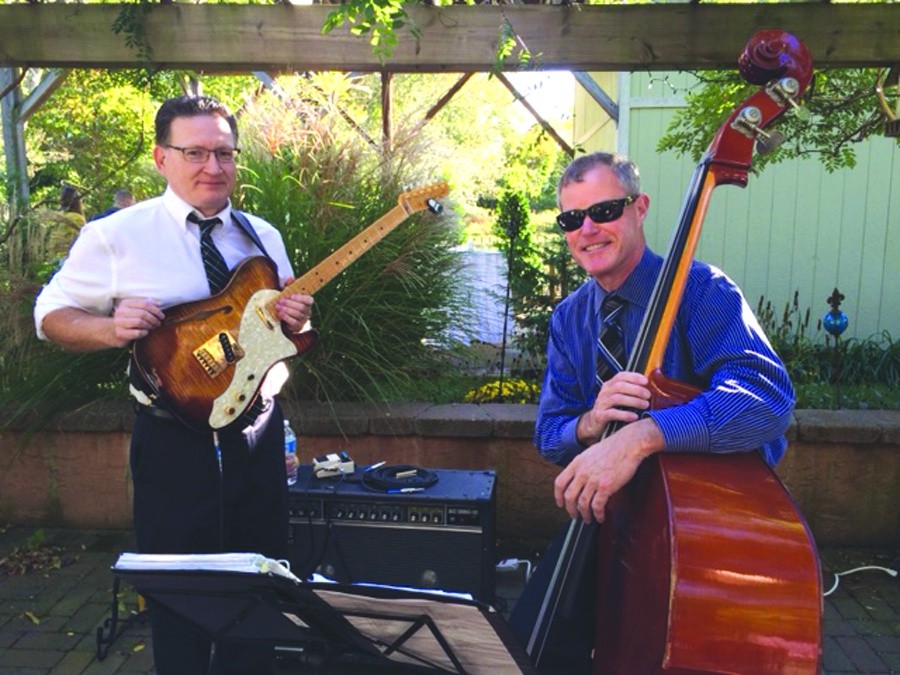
[522,28,824,675]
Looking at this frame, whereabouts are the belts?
[139,405,175,419]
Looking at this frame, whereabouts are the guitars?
[127,182,450,437]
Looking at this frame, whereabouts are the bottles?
[283,420,298,486]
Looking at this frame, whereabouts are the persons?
[57,184,135,265]
[503,149,796,674]
[34,93,315,675]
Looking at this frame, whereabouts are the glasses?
[164,143,240,164]
[556,195,636,232]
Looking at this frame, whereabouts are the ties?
[186,213,229,297]
[596,296,626,394]
